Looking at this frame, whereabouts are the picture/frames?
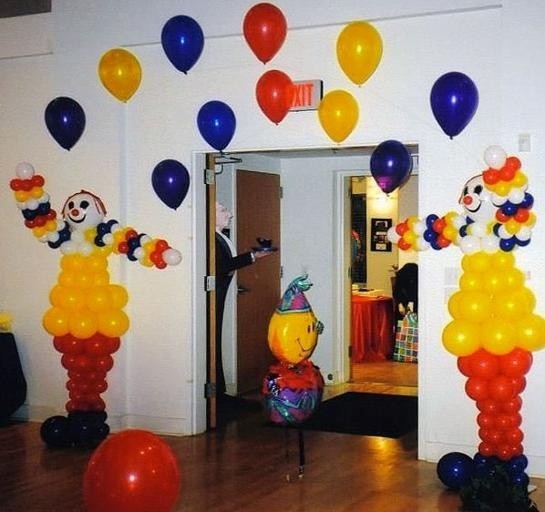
[369,216,392,254]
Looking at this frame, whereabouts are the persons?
[215,202,274,407]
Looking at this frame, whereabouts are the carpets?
[268,390,418,440]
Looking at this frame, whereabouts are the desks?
[350,294,395,364]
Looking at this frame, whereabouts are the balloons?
[262,273,325,477]
[44,96,86,152]
[98,49,142,104]
[196,100,236,152]
[430,72,479,141]
[84,429,181,512]
[369,141,413,195]
[318,89,360,145]
[161,15,204,74]
[255,70,294,126]
[243,1,287,65]
[336,20,383,87]
